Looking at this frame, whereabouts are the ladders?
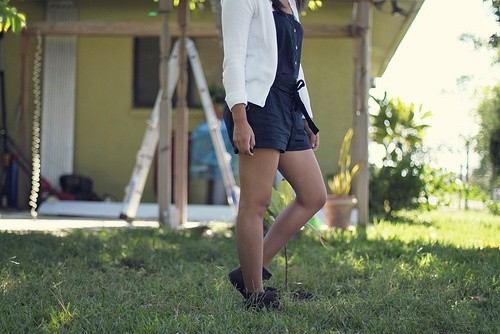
[117,36,244,224]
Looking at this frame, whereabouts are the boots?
[247,292,314,313]
[229,265,273,299]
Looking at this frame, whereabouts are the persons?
[221,0,327,312]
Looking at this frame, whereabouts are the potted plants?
[323,127,360,232]
